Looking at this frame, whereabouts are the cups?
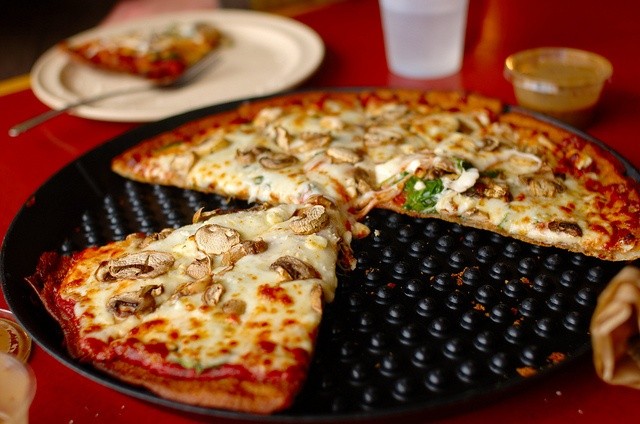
[382,3,466,78]
[506,49,611,123]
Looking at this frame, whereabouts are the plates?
[31,12,329,122]
[2,84,639,418]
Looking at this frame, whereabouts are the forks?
[10,55,220,137]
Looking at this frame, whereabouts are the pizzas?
[58,22,235,89]
[16,196,373,415]
[114,88,639,269]
